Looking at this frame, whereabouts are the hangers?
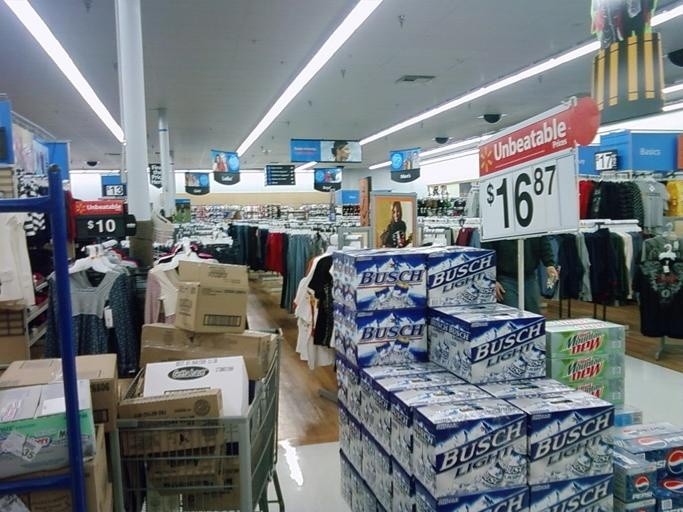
[68,243,117,274]
[658,243,675,261]
[662,222,677,242]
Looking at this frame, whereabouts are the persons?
[411,151,421,169]
[328,141,352,162]
[212,154,227,171]
[480,236,558,315]
[378,200,413,248]
[403,152,412,169]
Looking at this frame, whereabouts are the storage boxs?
[150,444,226,495]
[183,455,240,512]
[1,423,107,512]
[139,320,270,381]
[0,354,117,431]
[117,388,222,456]
[0,379,96,478]
[144,355,250,442]
[332,244,683,512]
[163,259,249,334]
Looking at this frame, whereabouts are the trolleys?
[111,328,285,512]
